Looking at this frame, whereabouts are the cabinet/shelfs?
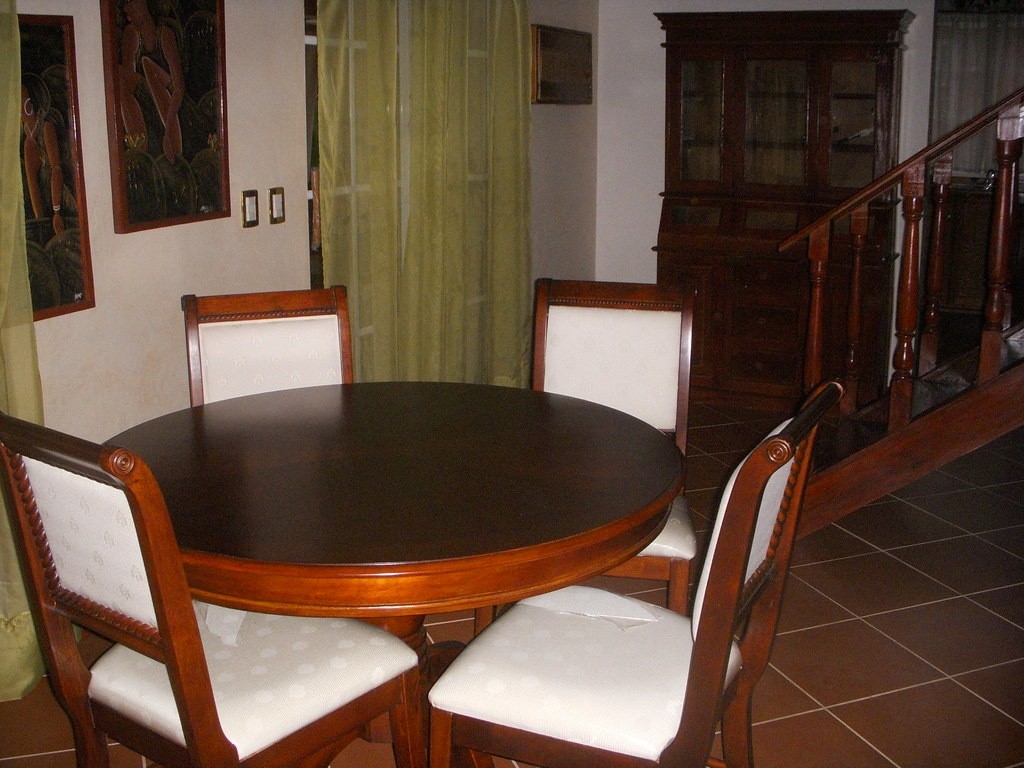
[651,8,917,413]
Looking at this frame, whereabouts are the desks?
[100,380,688,768]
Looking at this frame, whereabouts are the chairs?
[180,284,356,407]
[427,380,845,768]
[0,411,425,768]
[531,277,697,615]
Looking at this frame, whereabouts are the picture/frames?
[99,0,233,235]
[531,23,592,106]
[0,14,95,329]
